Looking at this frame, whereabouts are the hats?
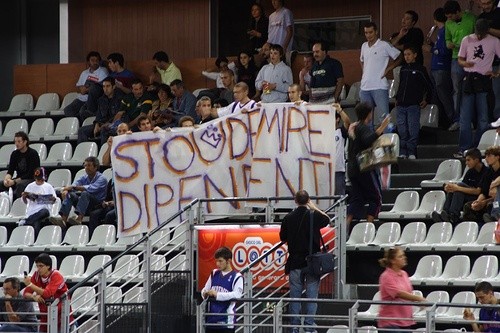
[491,118,500,127]
[33,168,44,178]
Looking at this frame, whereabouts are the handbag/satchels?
[308,251,335,275]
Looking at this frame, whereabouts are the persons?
[22,254,78,333]
[490,118,500,147]
[377,245,425,333]
[64,41,344,165]
[246,5,268,53]
[280,190,331,333]
[17,167,57,241]
[333,100,391,241]
[463,281,500,333]
[0,131,40,202]
[266,0,294,63]
[432,147,500,226]
[359,0,500,126]
[51,157,108,231]
[200,247,244,333]
[0,277,38,331]
[89,178,117,237]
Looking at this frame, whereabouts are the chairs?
[0,79,500,333]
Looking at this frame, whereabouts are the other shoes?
[68,217,79,225]
[49,216,65,229]
[432,211,453,222]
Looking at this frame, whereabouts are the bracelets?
[27,282,32,288]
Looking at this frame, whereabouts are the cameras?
[24,271,27,278]
